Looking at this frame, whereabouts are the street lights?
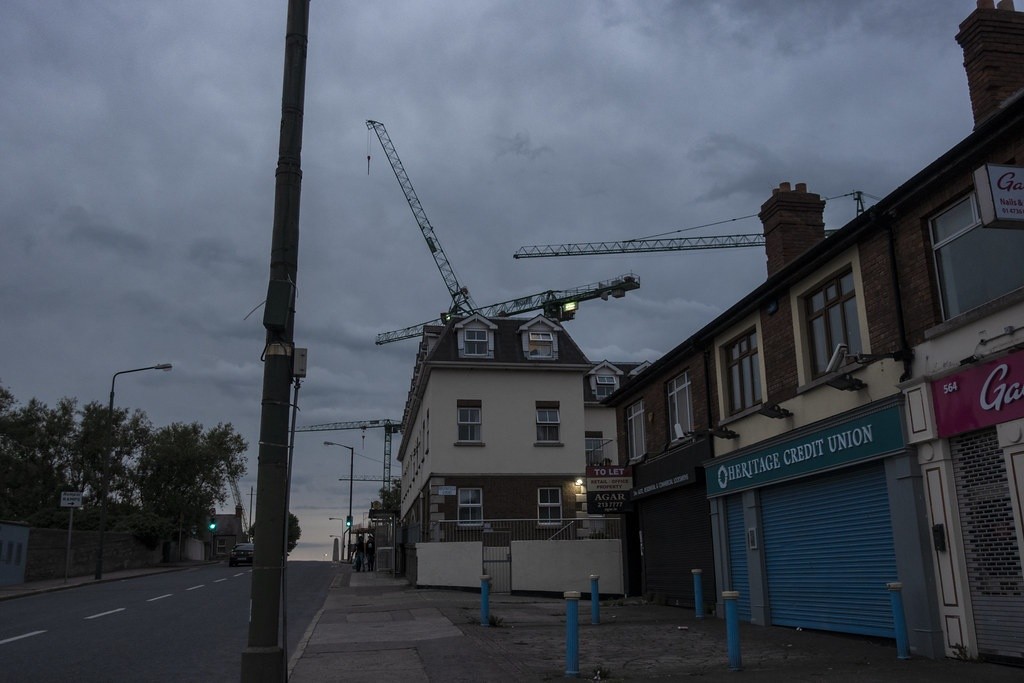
[322,440,355,561]
[94,361,173,580]
[328,517,344,537]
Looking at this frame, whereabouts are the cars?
[229,543,254,568]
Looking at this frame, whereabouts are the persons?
[364,531,376,571]
[352,535,365,572]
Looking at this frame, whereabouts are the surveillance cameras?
[825,343,847,374]
[674,423,685,440]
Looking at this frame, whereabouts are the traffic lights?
[209,518,218,532]
[345,516,352,530]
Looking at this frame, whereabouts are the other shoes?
[371,569,373,571]
[363,569,365,572]
[368,569,370,571]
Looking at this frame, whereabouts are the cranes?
[511,189,883,261]
[367,117,642,345]
[338,472,403,482]
[288,418,403,504]
